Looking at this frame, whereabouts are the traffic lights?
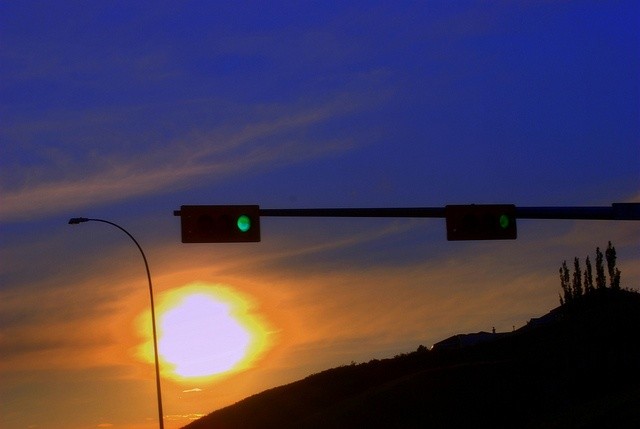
[445,204,518,240]
[181,204,261,243]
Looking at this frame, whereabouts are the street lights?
[67,217,164,429]
